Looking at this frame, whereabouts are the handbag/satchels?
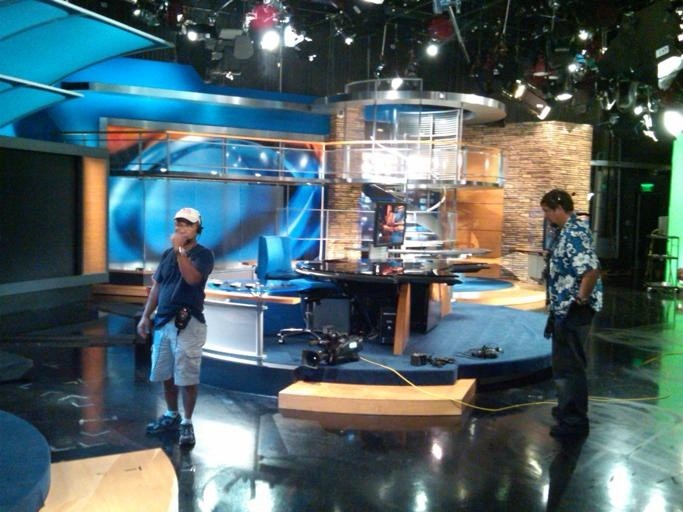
[175,306,191,336]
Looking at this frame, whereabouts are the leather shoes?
[549,405,589,438]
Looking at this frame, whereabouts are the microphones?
[187,233,199,243]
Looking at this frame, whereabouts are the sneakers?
[144,414,195,447]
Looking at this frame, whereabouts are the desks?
[295,260,490,357]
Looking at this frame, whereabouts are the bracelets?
[174,247,185,255]
[576,295,590,305]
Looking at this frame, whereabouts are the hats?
[173,208,201,223]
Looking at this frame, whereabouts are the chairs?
[255,236,302,336]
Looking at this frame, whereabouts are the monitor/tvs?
[374,202,406,247]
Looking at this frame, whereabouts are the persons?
[376,203,404,244]
[135,207,216,445]
[538,188,604,438]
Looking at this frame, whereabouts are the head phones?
[197,210,203,233]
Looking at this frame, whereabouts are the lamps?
[502,22,683,149]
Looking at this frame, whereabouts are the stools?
[277,280,339,346]
